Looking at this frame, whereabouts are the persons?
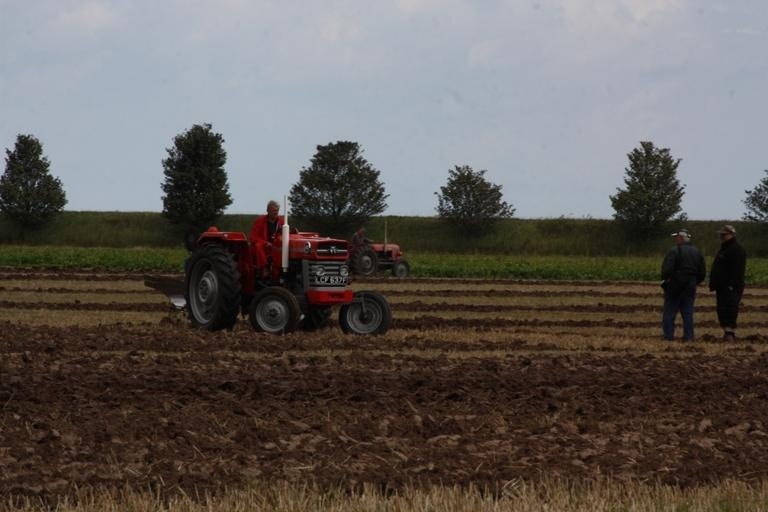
[352,229,374,247]
[250,200,284,281]
[708,224,746,340]
[660,228,706,341]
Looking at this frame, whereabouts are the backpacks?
[673,245,704,289]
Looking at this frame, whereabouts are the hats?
[670,227,691,242]
[714,224,736,235]
[267,201,279,212]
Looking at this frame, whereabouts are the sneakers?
[721,330,737,344]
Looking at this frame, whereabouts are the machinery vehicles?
[350,220,410,281]
[142,195,394,337]
